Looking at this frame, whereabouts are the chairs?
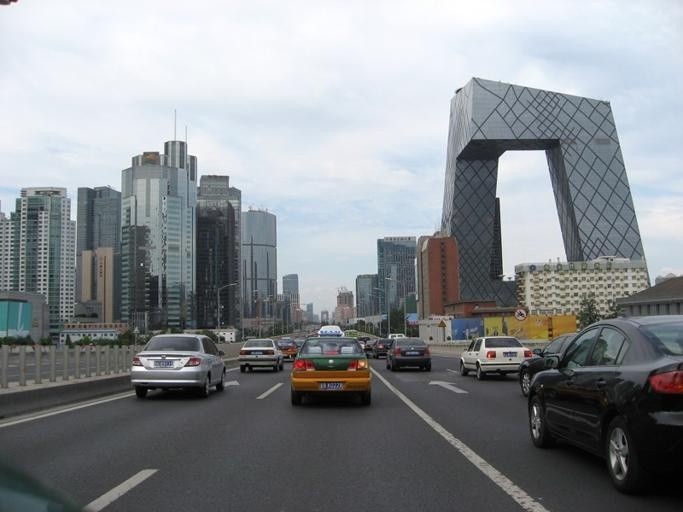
[307,345,353,354]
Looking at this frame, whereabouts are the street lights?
[216,282,291,342]
[342,276,408,339]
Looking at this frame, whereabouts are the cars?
[366,341,374,352]
[389,334,406,338]
[129,333,227,398]
[526,315,682,494]
[458,335,532,380]
[515,333,582,399]
[293,337,306,349]
[238,339,284,372]
[371,338,394,359]
[386,337,433,373]
[288,325,373,407]
[276,337,298,360]
